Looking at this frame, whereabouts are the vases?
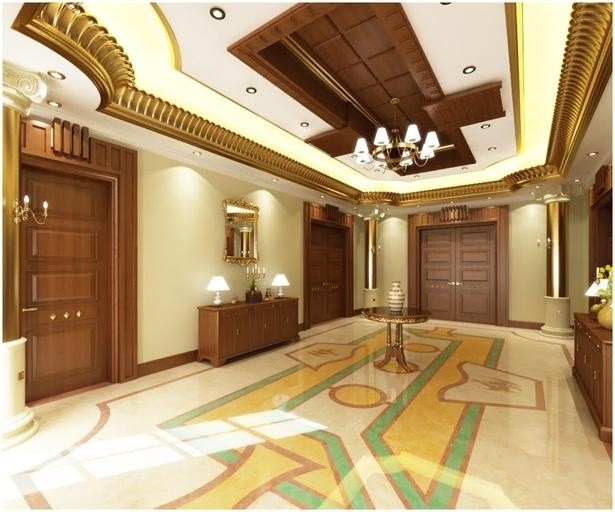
[597,296,613,331]
[385,280,407,314]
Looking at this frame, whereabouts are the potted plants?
[243,264,264,303]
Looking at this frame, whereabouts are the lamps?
[11,192,50,229]
[269,271,293,298]
[348,96,444,177]
[205,274,231,307]
[536,236,552,251]
[583,278,610,318]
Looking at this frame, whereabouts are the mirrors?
[221,195,260,269]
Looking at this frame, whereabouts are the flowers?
[596,264,614,300]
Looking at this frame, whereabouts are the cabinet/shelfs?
[571,312,612,443]
[194,296,300,367]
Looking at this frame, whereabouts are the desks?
[361,304,432,375]
